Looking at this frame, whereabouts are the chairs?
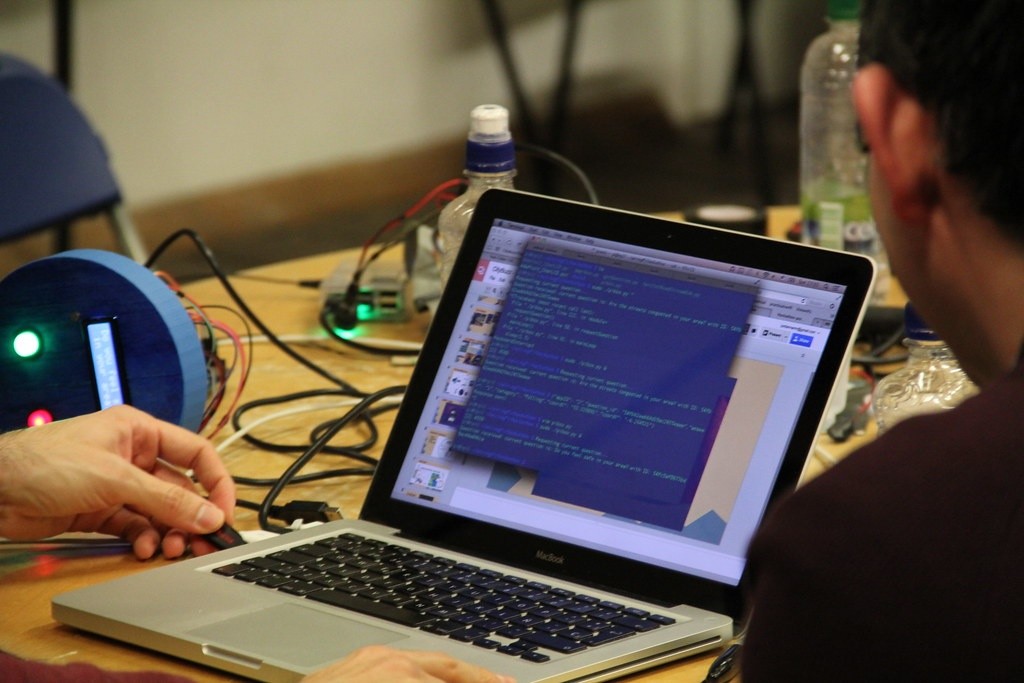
[0,50,151,271]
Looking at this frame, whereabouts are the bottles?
[438,103,516,301]
[803,1,890,308]
[873,300,976,439]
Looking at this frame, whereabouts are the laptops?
[51,187,876,683]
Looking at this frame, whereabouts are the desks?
[0,201,917,683]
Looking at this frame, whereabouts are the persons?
[2,400,578,680]
[704,1,1023,683]
